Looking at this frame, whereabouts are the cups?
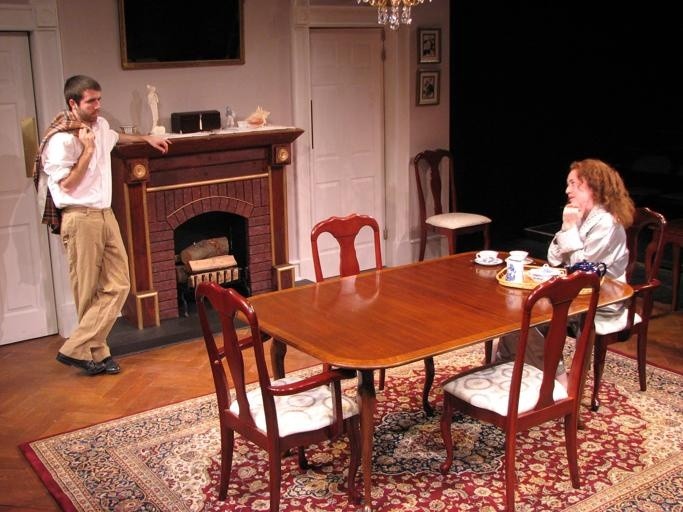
[119,125,136,133]
[506,261,524,282]
[476,250,499,263]
[236,121,247,130]
[526,266,561,284]
[508,251,528,261]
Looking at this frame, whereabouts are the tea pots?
[565,260,606,284]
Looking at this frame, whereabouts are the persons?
[489,157,639,432]
[32,75,173,374]
[423,35,435,97]
[224,106,237,128]
[145,84,160,126]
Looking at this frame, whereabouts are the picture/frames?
[415,68,440,106]
[114,1,247,71]
[415,26,441,66]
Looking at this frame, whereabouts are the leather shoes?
[100,357,120,374]
[56,352,105,375]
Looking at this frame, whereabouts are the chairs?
[191,277,362,510]
[587,205,667,413]
[308,209,435,426]
[441,270,602,511]
[405,146,495,263]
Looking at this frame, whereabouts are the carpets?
[13,334,681,510]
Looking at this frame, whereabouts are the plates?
[505,255,535,265]
[473,257,504,266]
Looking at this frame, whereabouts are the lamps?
[356,0,431,36]
